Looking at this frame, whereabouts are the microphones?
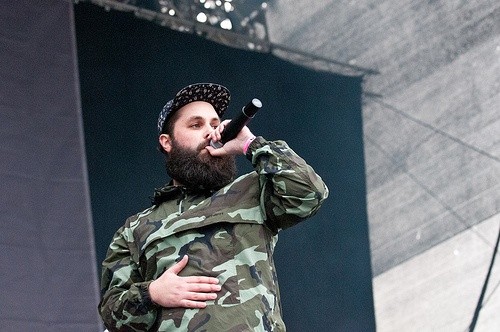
[210,98,262,149]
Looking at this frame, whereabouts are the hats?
[157,82,231,151]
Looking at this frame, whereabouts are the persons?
[95,83,332,332]
